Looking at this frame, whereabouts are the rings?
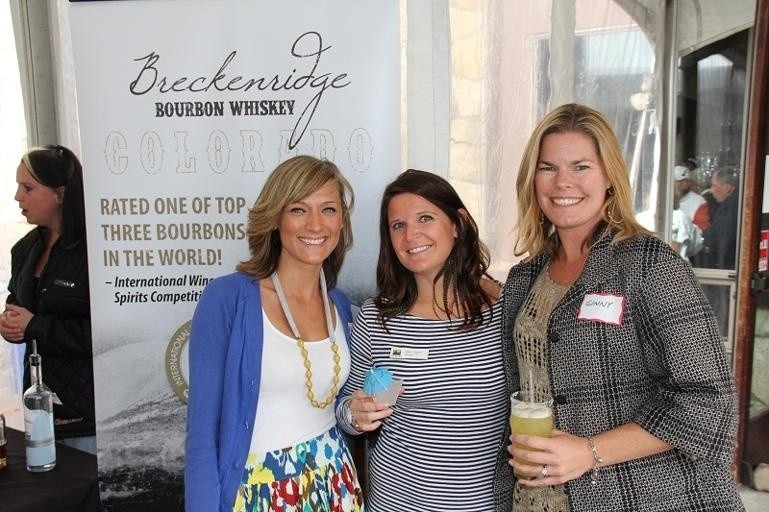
[542,465,549,479]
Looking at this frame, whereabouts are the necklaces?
[270,264,341,411]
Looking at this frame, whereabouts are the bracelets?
[340,399,362,434]
[586,434,604,486]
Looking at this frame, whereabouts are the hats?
[675,165,690,181]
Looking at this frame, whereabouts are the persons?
[333,167,520,512]
[180,152,373,512]
[496,101,752,512]
[0,143,98,457]
[634,156,741,340]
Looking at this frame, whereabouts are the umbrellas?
[363,366,393,396]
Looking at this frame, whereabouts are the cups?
[508,389,555,481]
[372,377,404,406]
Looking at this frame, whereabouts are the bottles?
[19,353,58,474]
